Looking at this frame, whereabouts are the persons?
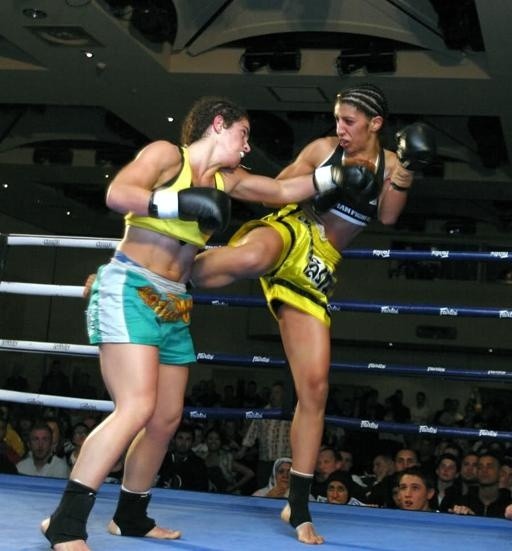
[84,83,437,545]
[2,358,511,521]
[39,93,386,551]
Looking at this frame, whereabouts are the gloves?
[313,162,378,200]
[149,186,231,236]
[396,122,432,170]
